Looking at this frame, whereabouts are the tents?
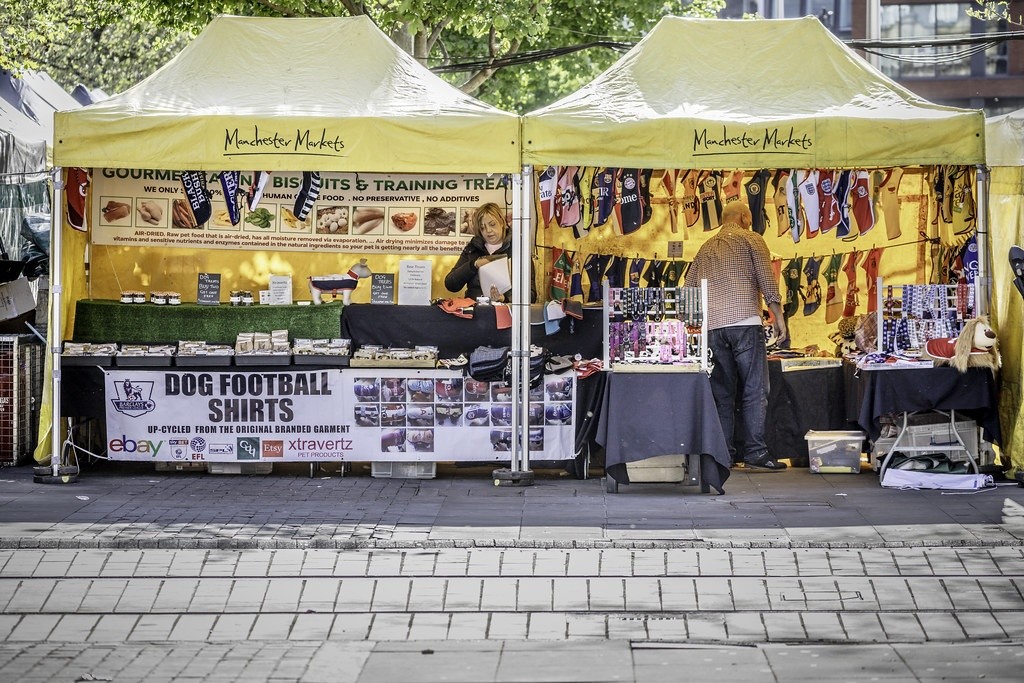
[0,65,113,480]
[978,106,1024,489]
[27,11,518,490]
[524,14,988,490]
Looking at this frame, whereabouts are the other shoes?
[745,447,787,471]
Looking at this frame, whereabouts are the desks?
[74,296,343,343]
[57,361,584,478]
[734,354,847,467]
[839,354,992,440]
[338,303,549,353]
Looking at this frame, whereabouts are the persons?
[445,202,516,303]
[682,200,791,473]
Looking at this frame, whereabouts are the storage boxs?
[0,333,49,468]
[0,276,37,323]
[894,409,980,464]
[804,429,865,474]
